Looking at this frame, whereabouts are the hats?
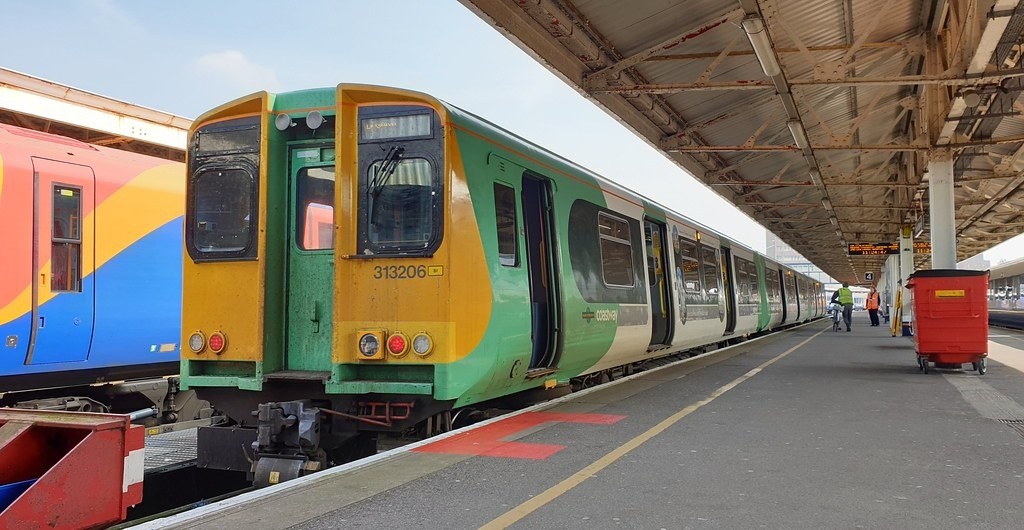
[842,282,848,287]
[870,285,875,288]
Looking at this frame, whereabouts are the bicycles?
[830,299,845,332]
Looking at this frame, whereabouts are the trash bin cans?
[904,269,991,375]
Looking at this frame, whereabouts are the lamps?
[809,167,826,191]
[962,89,980,107]
[840,239,847,246]
[741,12,780,77]
[821,197,833,211]
[829,216,838,226]
[785,118,808,149]
[835,229,842,237]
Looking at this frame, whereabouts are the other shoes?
[847,328,851,331]
[871,322,880,326]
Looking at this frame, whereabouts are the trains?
[178,83,827,492]
[0,122,223,429]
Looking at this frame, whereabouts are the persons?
[832,282,854,332]
[866,285,881,327]
